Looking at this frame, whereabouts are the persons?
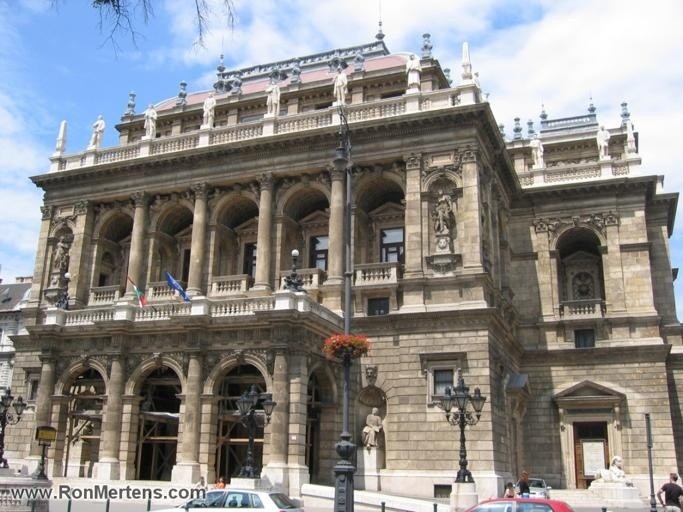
[144,104,157,138]
[194,476,208,491]
[91,114,105,147]
[54,235,68,270]
[203,93,217,125]
[504,470,530,498]
[435,189,453,233]
[530,134,544,165]
[332,66,348,102]
[406,54,422,89]
[656,473,683,512]
[363,407,382,450]
[216,476,226,489]
[596,125,610,156]
[265,79,280,114]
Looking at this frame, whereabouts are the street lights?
[328,104,359,512]
[0,388,27,470]
[236,384,277,479]
[435,368,486,483]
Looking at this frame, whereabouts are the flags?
[165,270,191,303]
[132,285,149,309]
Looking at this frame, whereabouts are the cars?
[511,476,551,499]
[462,496,573,512]
[151,487,305,512]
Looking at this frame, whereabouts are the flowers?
[323,334,372,363]
